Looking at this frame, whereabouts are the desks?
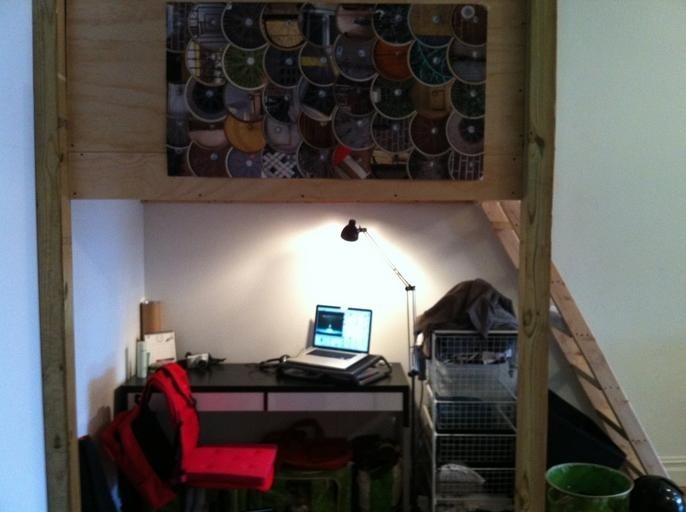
[121,362,410,512]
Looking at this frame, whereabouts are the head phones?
[256,355,289,371]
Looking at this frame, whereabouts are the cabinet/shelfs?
[422,327,518,512]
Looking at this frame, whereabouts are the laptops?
[284,304,372,371]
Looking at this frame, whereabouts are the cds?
[168,0,486,181]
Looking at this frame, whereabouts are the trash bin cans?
[545,463,634,512]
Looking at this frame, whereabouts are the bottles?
[136,342,147,378]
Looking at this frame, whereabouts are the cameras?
[186,354,210,369]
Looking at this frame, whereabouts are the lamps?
[339,219,418,511]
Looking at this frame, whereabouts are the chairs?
[150,363,279,512]
